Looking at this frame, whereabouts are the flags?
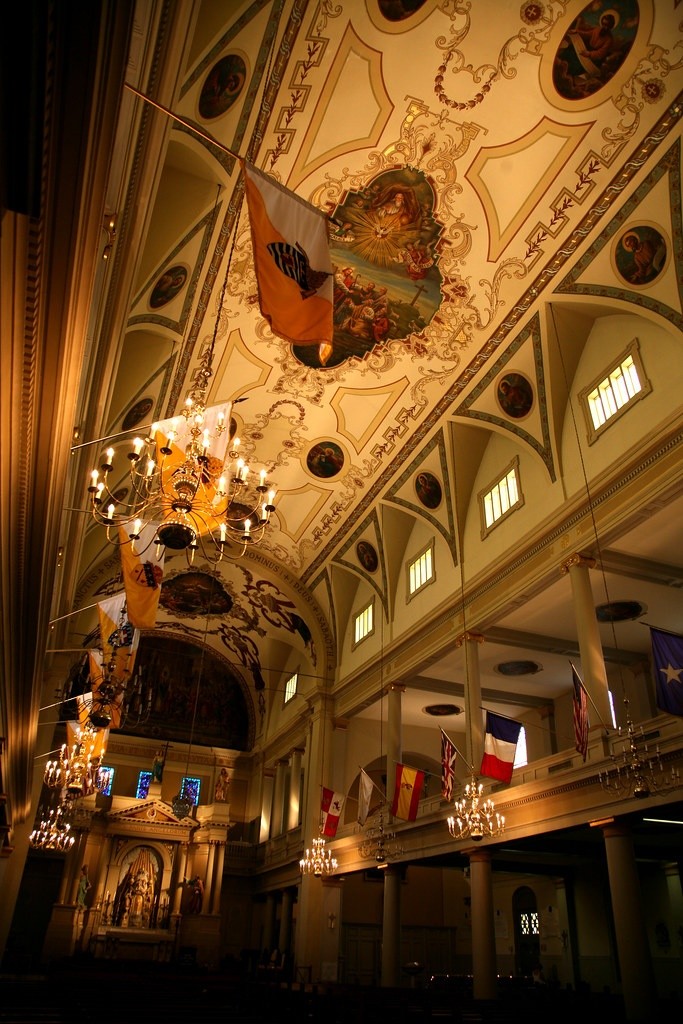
[649,626,683,717]
[571,667,588,763]
[98,592,141,683]
[480,712,522,784]
[66,646,124,771]
[116,515,165,631]
[391,763,425,822]
[357,770,374,827]
[440,729,456,802]
[320,787,347,839]
[240,158,335,366]
[151,401,231,539]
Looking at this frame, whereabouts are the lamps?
[27,795,76,853]
[357,503,407,863]
[82,181,276,567]
[172,604,212,822]
[298,562,340,877]
[51,594,157,730]
[446,420,507,842]
[547,302,682,802]
[39,725,110,797]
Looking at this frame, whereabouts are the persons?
[153,750,164,783]
[77,864,92,906]
[183,873,205,914]
[217,767,229,802]
